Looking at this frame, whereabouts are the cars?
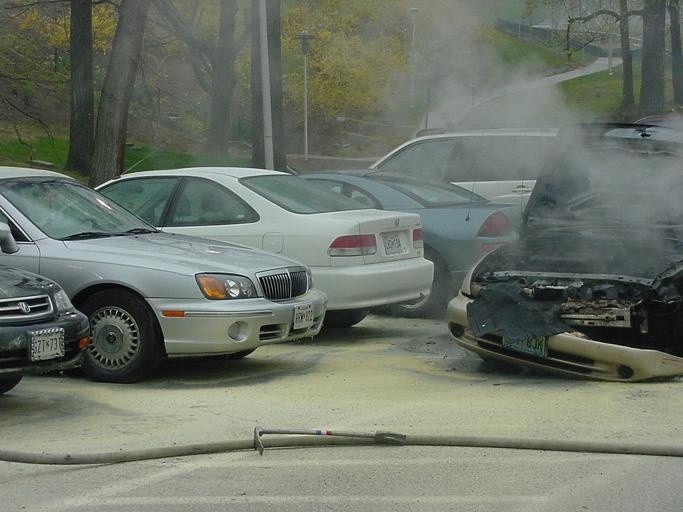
[0,265,90,393]
[0,165,326,384]
[302,170,523,316]
[40,166,435,327]
[447,122,683,383]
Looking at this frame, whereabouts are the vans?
[371,126,559,217]
[609,115,683,144]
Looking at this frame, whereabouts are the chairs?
[197,190,237,221]
[166,190,193,224]
